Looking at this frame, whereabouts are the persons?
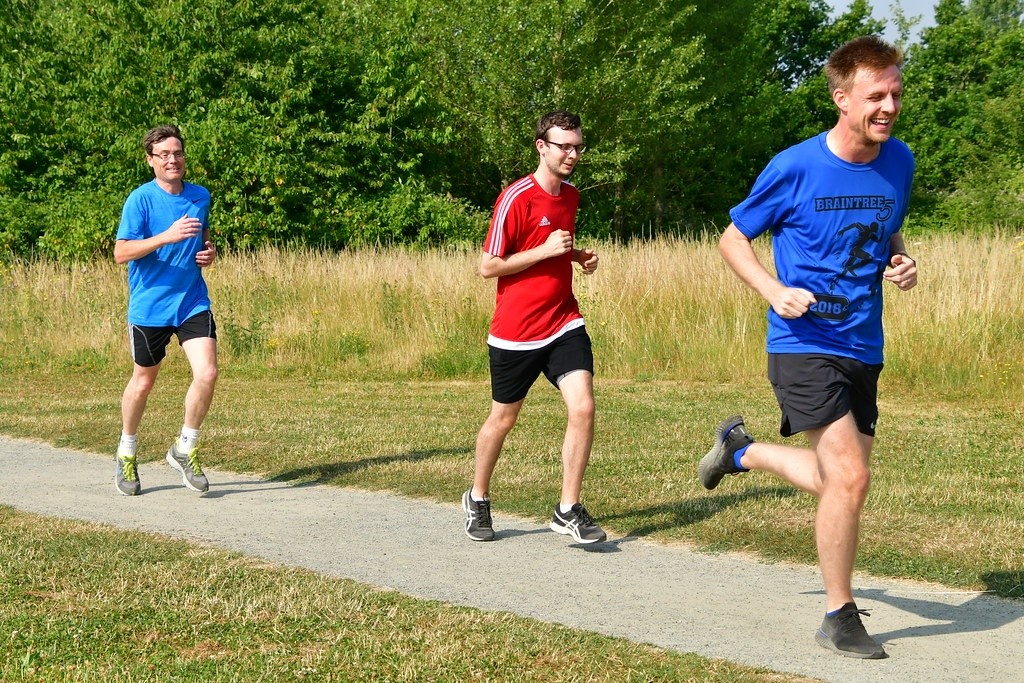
[697,37,917,660]
[115,126,217,496]
[460,109,606,543]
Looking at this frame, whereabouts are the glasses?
[152,152,186,162]
[546,140,587,154]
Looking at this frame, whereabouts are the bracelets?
[898,251,917,268]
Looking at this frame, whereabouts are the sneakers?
[550,502,606,544]
[116,452,141,495]
[699,415,753,490]
[816,603,885,659]
[462,486,495,541]
[166,441,209,491]
